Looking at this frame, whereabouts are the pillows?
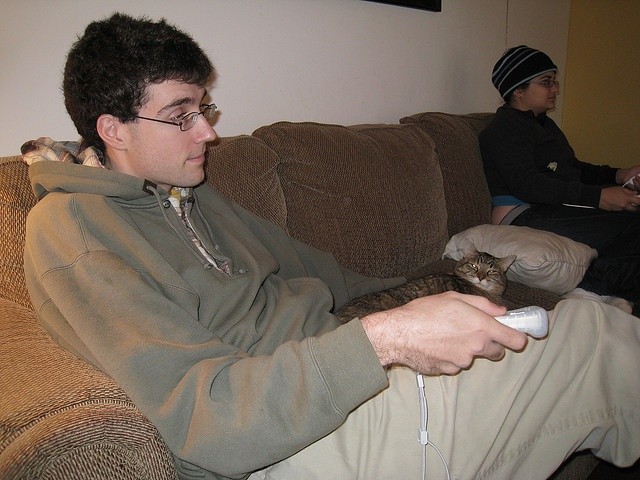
[442,222,597,294]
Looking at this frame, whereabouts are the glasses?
[526,80,559,88]
[114,104,217,132]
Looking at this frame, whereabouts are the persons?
[479,45,640,316]
[22,11,640,480]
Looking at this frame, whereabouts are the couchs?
[0,112,598,480]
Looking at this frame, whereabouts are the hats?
[491,45,558,102]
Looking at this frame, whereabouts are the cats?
[332,237,518,324]
